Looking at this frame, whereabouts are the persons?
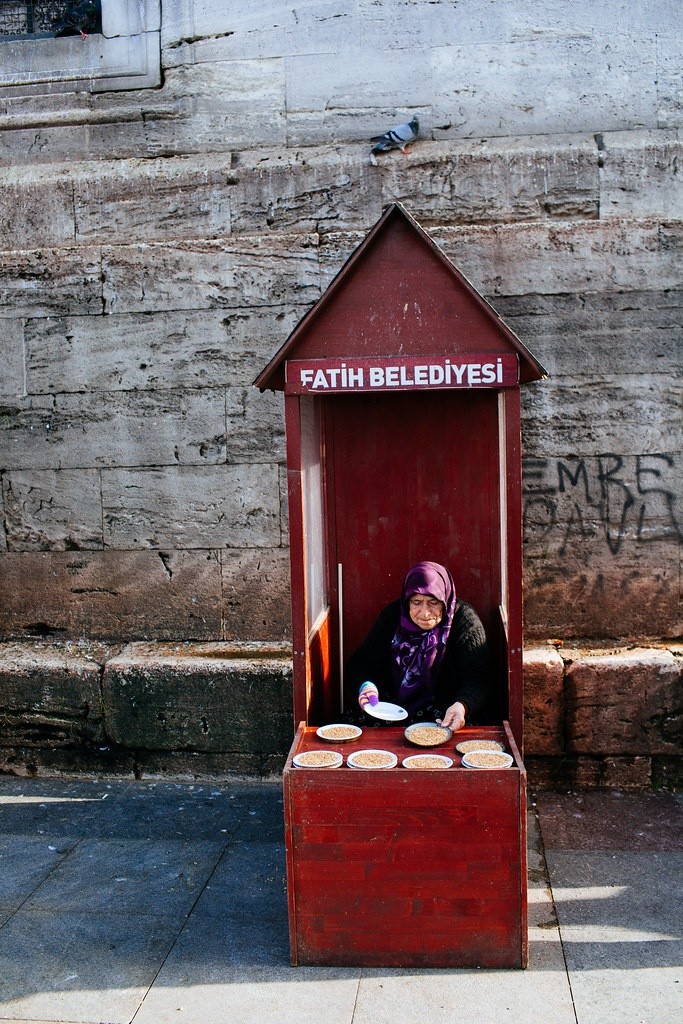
[340,561,492,731]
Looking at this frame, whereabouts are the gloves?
[359,681,379,709]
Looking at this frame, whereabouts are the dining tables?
[282,720,529,971]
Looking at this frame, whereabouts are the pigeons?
[368,113,420,157]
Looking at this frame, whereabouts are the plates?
[404,722,453,746]
[461,749,514,769]
[402,754,453,769]
[363,702,408,721]
[456,740,506,754]
[316,723,363,743]
[293,751,343,768]
[346,749,398,769]
